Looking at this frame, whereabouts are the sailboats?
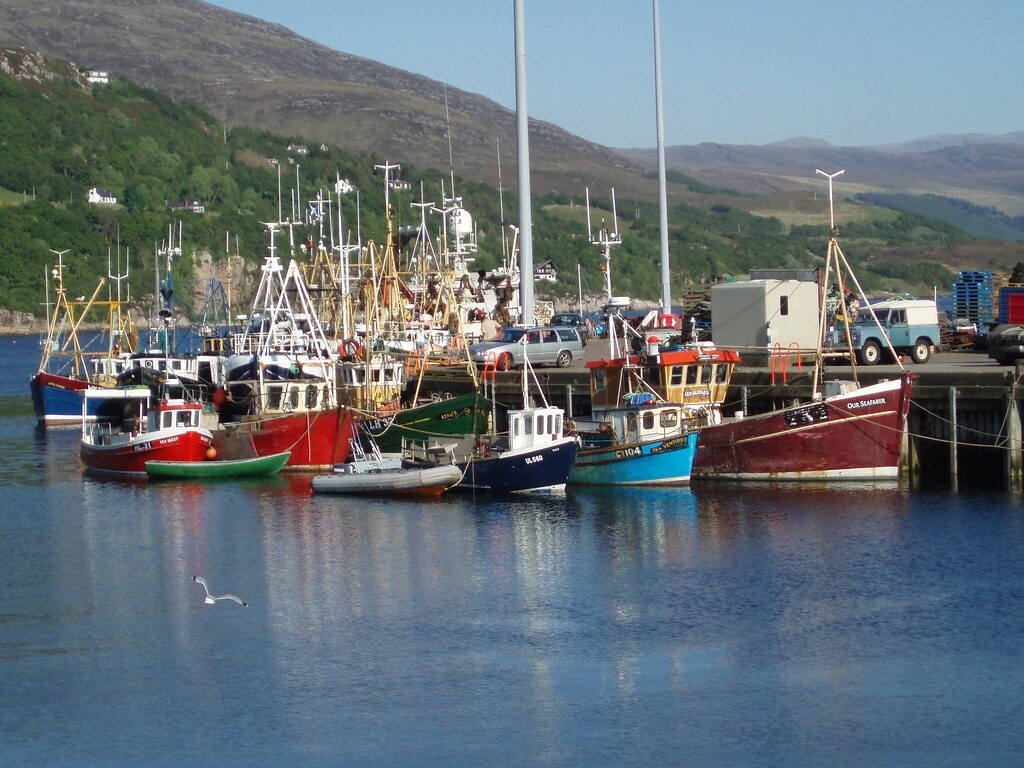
[198,279,211,338]
[38,308,60,352]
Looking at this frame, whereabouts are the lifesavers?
[306,387,317,400]
[339,338,361,359]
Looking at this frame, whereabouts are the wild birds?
[190,574,252,611]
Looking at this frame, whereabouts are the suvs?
[462,327,585,368]
[825,297,940,363]
[987,323,1024,366]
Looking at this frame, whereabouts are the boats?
[30,249,123,427]
[81,217,214,474]
[307,433,462,493]
[567,315,702,486]
[217,164,353,472]
[334,170,492,452]
[458,331,586,497]
[287,155,557,361]
[588,0,915,480]
[90,282,135,386]
[144,450,290,479]
[582,183,741,433]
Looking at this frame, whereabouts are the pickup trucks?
[547,312,587,346]
